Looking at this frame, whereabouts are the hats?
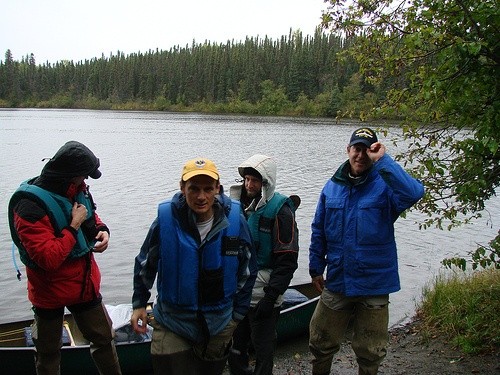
[89,168,102,178]
[182,157,220,183]
[349,127,378,150]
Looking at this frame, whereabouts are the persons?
[131,157,259,375]
[8,140,122,375]
[228,153,301,375]
[308,127,424,375]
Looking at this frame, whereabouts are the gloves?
[251,294,275,323]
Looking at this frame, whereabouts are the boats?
[0,275,327,375]
